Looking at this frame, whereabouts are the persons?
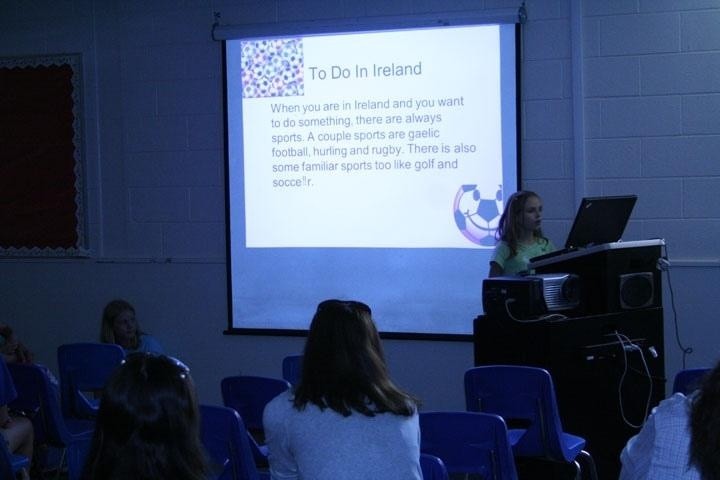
[75,350,214,479]
[0,403,34,480]
[487,186,555,279]
[0,325,57,386]
[618,362,719,480]
[90,298,163,401]
[262,297,425,480]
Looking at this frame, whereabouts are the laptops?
[529,194,638,261]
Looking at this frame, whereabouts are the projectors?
[482,273,583,318]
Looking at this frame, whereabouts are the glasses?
[119,351,190,381]
